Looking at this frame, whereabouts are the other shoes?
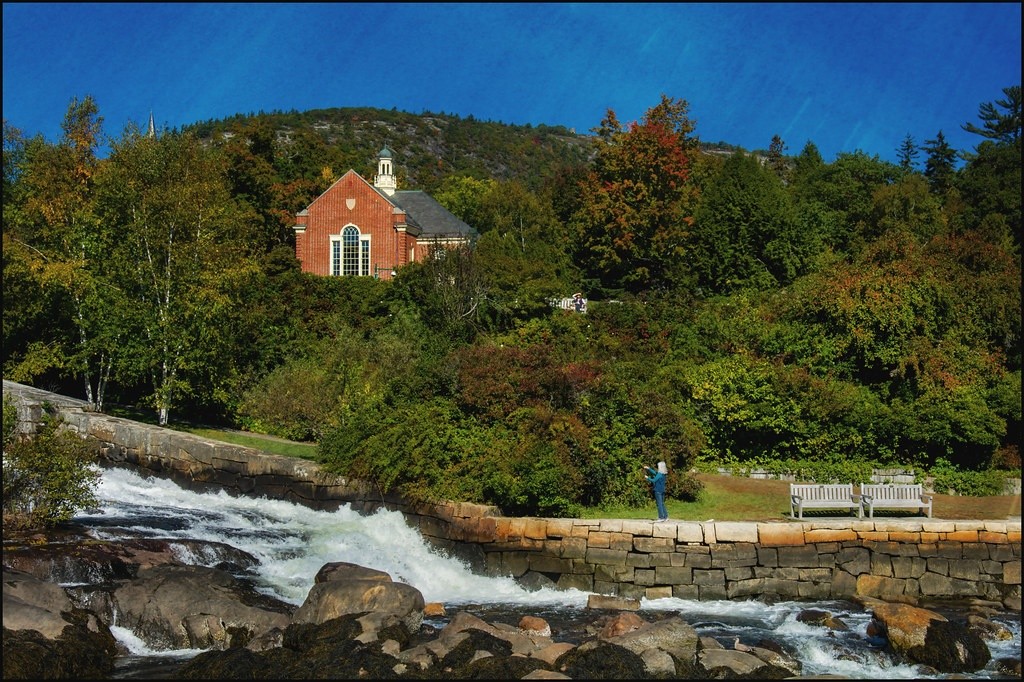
[655,519,670,523]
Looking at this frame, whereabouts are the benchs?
[790,483,863,520]
[860,483,933,519]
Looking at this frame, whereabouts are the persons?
[644,461,668,522]
[574,294,583,311]
